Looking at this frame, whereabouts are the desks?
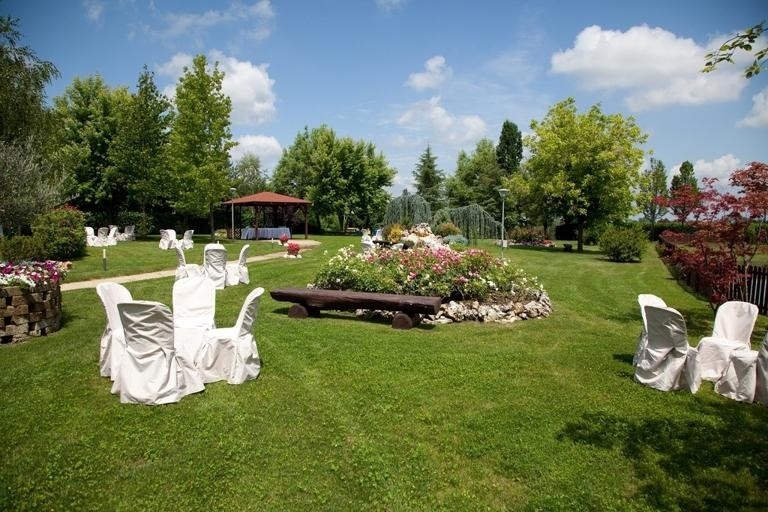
[240,228,291,240]
[373,240,394,249]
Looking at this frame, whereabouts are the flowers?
[0,257,73,287]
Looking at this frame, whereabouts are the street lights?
[497,188,510,259]
[230,188,237,239]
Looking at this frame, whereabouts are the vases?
[0,280,63,346]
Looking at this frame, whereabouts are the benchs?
[270,288,441,330]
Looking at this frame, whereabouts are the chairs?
[359,241,375,253]
[372,228,383,241]
[391,242,403,250]
[633,293,760,405]
[83,223,264,405]
[278,225,287,228]
